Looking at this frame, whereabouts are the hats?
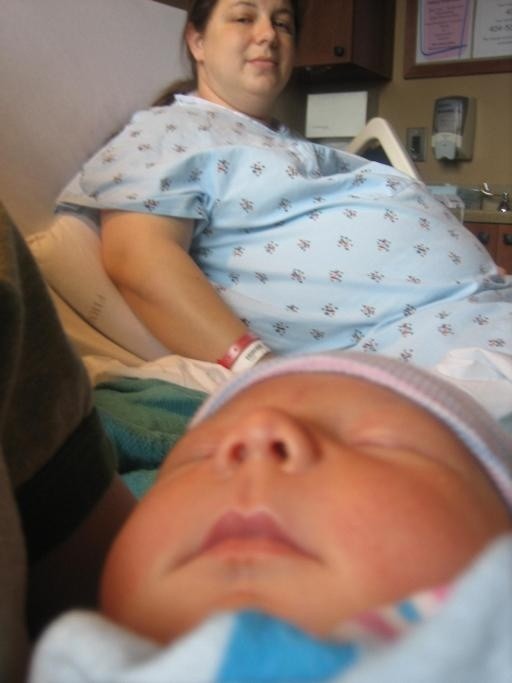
[187,350,512,507]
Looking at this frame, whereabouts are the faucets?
[480,188,510,212]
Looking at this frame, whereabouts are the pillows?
[16,204,180,366]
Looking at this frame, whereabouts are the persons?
[26,348,512,681]
[51,0,512,442]
[0,200,140,680]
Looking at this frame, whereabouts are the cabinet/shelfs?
[462,214,511,276]
[293,0,393,80]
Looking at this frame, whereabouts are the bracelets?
[229,339,272,374]
[217,328,262,369]
